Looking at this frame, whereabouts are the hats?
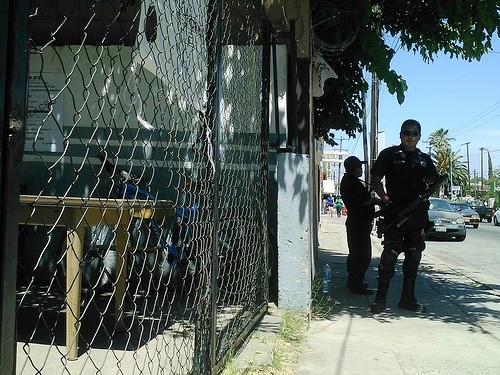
[344,156,367,169]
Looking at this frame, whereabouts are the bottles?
[322,261,332,294]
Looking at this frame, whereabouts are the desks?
[19,195,178,361]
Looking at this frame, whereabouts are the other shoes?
[347,275,369,293]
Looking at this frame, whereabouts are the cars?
[423,198,466,241]
[492,208,500,227]
[450,201,480,229]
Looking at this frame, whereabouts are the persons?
[340,156,386,294]
[369,119,441,313]
[323,193,343,218]
[429,203,434,209]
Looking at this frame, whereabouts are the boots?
[371,277,425,314]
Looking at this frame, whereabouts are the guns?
[377,174,448,238]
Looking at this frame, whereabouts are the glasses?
[402,130,419,137]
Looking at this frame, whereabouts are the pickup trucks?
[464,200,492,223]
